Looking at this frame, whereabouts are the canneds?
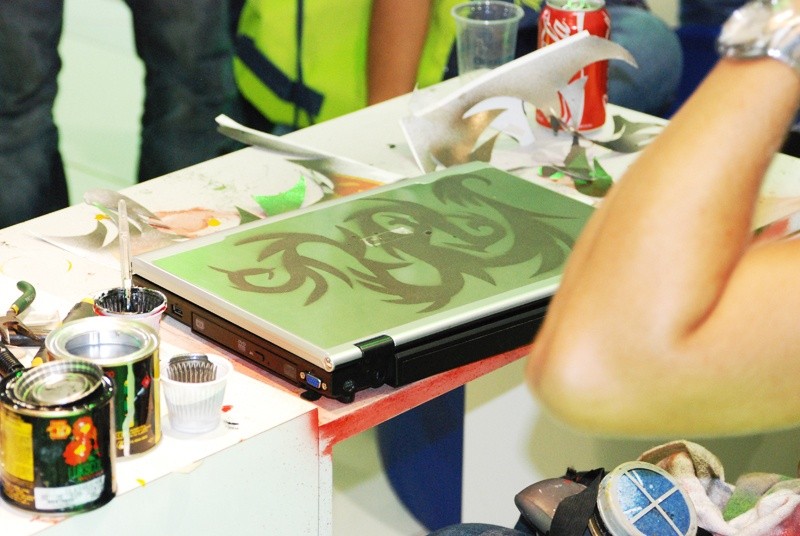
[0,315,161,514]
[535,0,612,132]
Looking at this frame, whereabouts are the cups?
[451,0,525,75]
[92,285,168,335]
[156,352,234,433]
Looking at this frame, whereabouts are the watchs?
[714,0,800,74]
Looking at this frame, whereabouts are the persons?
[526,0,800,439]
[0,0,683,229]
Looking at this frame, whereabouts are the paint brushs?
[117,199,135,312]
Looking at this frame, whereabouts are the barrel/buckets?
[0,317,163,517]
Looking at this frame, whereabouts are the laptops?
[126,160,594,405]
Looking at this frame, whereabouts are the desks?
[0,72,800,536]
